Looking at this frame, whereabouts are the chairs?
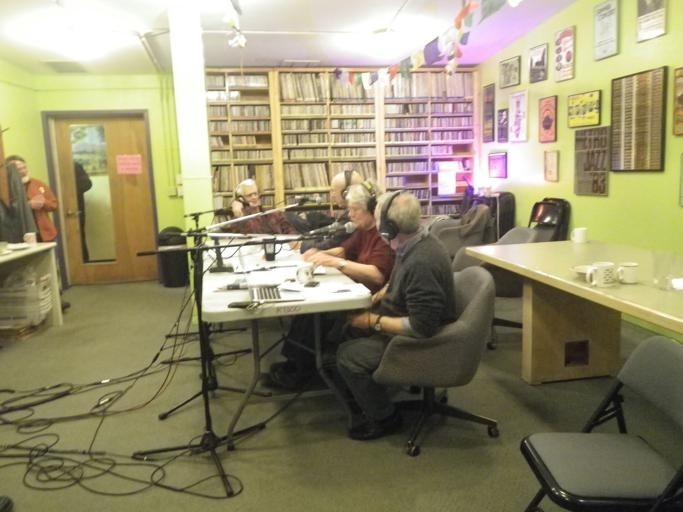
[428,203,491,259]
[524,197,571,241]
[515,333,682,512]
[368,264,501,460]
[449,223,557,350]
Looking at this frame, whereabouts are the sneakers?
[348,412,402,441]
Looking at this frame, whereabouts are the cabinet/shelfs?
[272,65,382,211]
[203,68,278,223]
[378,67,477,219]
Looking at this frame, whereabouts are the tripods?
[131,203,323,497]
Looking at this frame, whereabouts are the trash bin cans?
[157,226,188,288]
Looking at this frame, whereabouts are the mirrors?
[66,123,117,263]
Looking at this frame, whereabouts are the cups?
[584,261,616,289]
[651,248,674,290]
[22,232,36,246]
[296,265,313,283]
[617,262,638,282]
[477,185,491,197]
[262,237,281,261]
[570,228,588,243]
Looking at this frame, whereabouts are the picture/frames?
[487,151,507,179]
[543,149,559,183]
[609,65,669,173]
[634,0,668,46]
[593,0,619,62]
[571,125,611,197]
[481,82,495,143]
[672,66,683,138]
[494,23,601,145]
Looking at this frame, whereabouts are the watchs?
[374,314,383,332]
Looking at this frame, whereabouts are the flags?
[336,1,510,81]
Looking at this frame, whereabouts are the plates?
[7,243,27,250]
[568,264,591,277]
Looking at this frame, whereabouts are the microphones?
[315,221,355,235]
[298,193,320,205]
[293,196,322,203]
[308,222,341,236]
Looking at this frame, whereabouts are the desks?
[461,238,682,387]
[198,236,373,453]
[0,241,63,330]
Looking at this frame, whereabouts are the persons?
[301,169,363,255]
[74,160,92,263]
[335,189,455,441]
[257,181,397,392]
[229,179,298,234]
[7,154,70,312]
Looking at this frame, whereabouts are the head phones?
[234,191,249,209]
[340,171,352,199]
[377,188,406,240]
[359,181,377,214]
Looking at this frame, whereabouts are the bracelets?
[338,259,347,271]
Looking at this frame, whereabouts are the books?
[280,72,376,205]
[207,74,274,209]
[0,237,53,341]
[228,277,285,291]
[385,71,476,215]
